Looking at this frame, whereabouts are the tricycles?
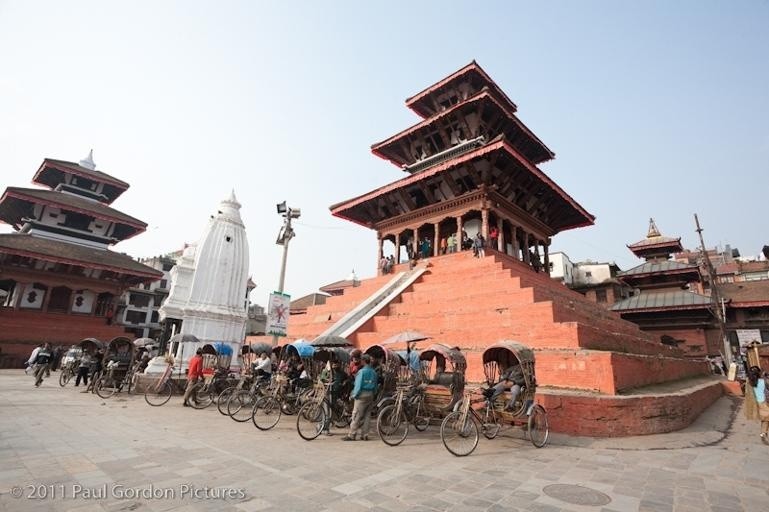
[59,335,157,399]
[143,330,433,439]
[439,340,550,457]
[378,342,467,446]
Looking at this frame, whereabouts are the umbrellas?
[167,332,200,365]
[307,333,353,361]
[379,328,433,371]
[133,337,154,350]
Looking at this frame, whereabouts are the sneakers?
[760,432,769,445]
[360,436,368,441]
[341,436,356,440]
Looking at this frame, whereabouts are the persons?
[280,350,303,398]
[340,354,379,441]
[23,341,155,387]
[369,354,383,416]
[315,360,352,435]
[347,348,364,375]
[182,347,205,407]
[250,350,272,386]
[277,354,290,373]
[710,337,769,444]
[478,363,527,411]
[380,226,499,276]
[105,306,115,325]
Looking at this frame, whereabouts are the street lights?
[273,200,302,346]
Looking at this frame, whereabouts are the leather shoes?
[504,405,514,412]
[481,386,495,394]
